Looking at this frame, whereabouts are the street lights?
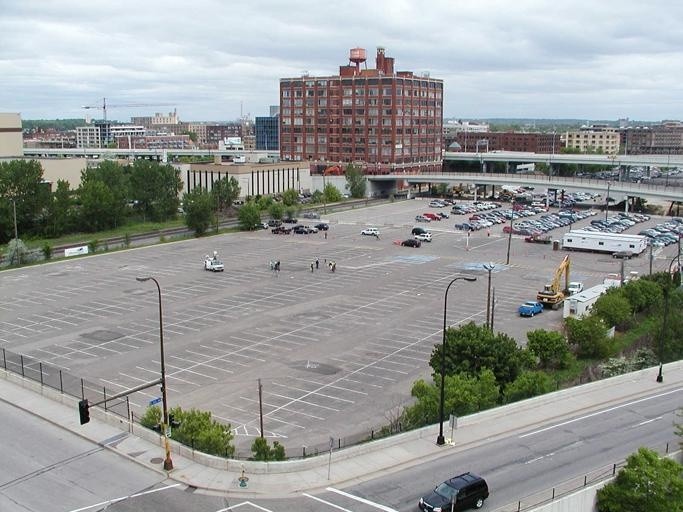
[550,128,557,157]
[645,242,653,281]
[481,261,494,331]
[130,274,174,471]
[434,275,477,447]
[616,125,657,156]
[4,194,21,265]
[620,258,627,289]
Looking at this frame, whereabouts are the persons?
[270,260,280,271]
[311,259,336,273]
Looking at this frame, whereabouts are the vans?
[401,239,420,248]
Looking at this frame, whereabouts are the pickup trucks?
[518,300,543,318]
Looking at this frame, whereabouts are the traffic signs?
[149,397,162,406]
[168,412,175,429]
[164,426,173,439]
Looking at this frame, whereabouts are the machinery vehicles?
[536,252,572,311]
[625,270,639,285]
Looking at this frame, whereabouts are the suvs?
[418,471,491,512]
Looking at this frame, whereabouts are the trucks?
[561,273,628,323]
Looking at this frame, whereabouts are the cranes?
[82,97,180,123]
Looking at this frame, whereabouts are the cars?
[217,187,324,210]
[251,212,331,237]
[201,257,226,271]
[567,280,584,295]
[361,226,379,235]
[407,165,681,265]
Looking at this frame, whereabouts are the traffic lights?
[170,421,180,432]
[152,423,162,432]
[78,399,92,425]
